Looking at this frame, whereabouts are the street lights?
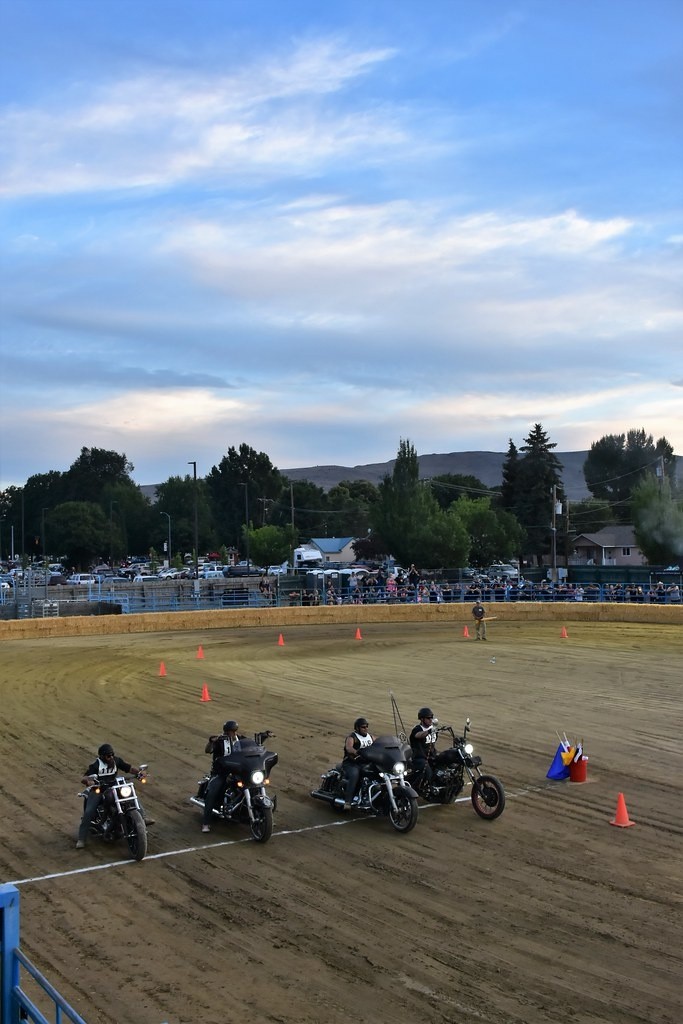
[188,462,199,579]
[41,507,49,556]
[110,500,119,552]
[159,512,171,566]
[237,483,250,577]
[287,480,295,537]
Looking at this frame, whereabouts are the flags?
[546,741,583,780]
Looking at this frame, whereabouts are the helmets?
[98,744,113,754]
[354,718,369,728]
[418,708,434,720]
[222,720,238,731]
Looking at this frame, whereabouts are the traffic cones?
[196,645,205,659]
[200,684,212,702]
[462,625,470,637]
[277,634,285,646]
[355,628,362,639]
[158,662,167,677]
[559,626,568,638]
[608,792,636,829]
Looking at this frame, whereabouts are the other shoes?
[76,839,85,849]
[482,637,486,640]
[477,638,480,640]
[143,817,155,825]
[201,824,211,833]
[344,801,351,809]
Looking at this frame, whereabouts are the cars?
[308,560,402,579]
[92,555,284,583]
[0,561,67,589]
[65,574,96,586]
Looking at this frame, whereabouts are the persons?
[472,600,486,640]
[76,744,155,848]
[259,564,681,605]
[344,718,376,809]
[408,708,438,778]
[202,721,246,832]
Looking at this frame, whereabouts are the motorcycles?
[310,734,420,833]
[406,717,505,821]
[189,731,279,844]
[77,764,150,861]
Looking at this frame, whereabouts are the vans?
[487,564,521,581]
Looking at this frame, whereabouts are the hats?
[476,600,480,603]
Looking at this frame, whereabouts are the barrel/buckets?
[570,759,587,782]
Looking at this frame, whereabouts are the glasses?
[108,753,115,758]
[424,716,433,720]
[361,726,369,729]
